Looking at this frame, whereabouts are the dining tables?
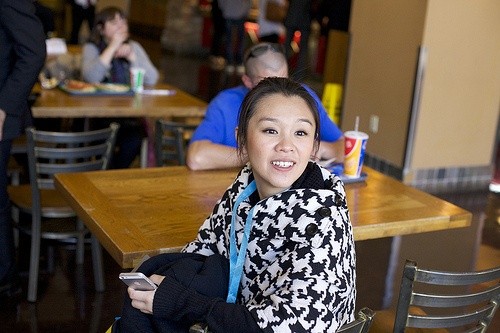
[9,78,203,177]
[55,140,472,313]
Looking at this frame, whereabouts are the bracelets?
[124,48,132,56]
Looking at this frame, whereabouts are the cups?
[343,131,369,178]
[129,67,145,93]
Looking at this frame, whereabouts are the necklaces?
[219,177,296,306]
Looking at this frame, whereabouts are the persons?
[280,0,317,82]
[69,1,99,43]
[219,1,253,74]
[208,1,229,66]
[78,4,161,167]
[256,1,284,42]
[95,75,359,333]
[0,0,52,333]
[185,39,349,170]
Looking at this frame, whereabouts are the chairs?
[4,118,123,298]
[357,259,500,333]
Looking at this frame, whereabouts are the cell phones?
[119,272,157,292]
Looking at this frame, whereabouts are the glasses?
[244,44,283,65]
